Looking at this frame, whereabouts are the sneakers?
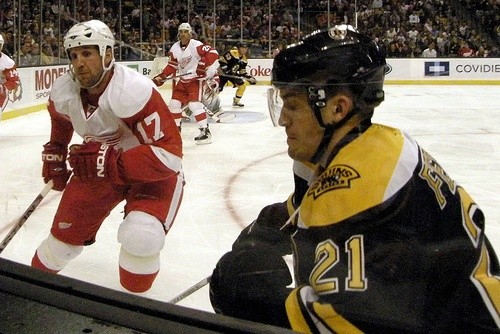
[233,97,245,108]
[194,128,212,145]
[237,41,249,49]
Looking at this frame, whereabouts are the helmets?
[64,21,114,79]
[267,25,390,126]
[177,23,192,32]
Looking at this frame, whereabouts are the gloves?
[67,140,113,181]
[41,145,71,191]
[231,203,293,255]
[209,252,292,317]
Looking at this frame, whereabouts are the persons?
[29,19,187,296]
[0,0,500,141]
[209,26,500,334]
